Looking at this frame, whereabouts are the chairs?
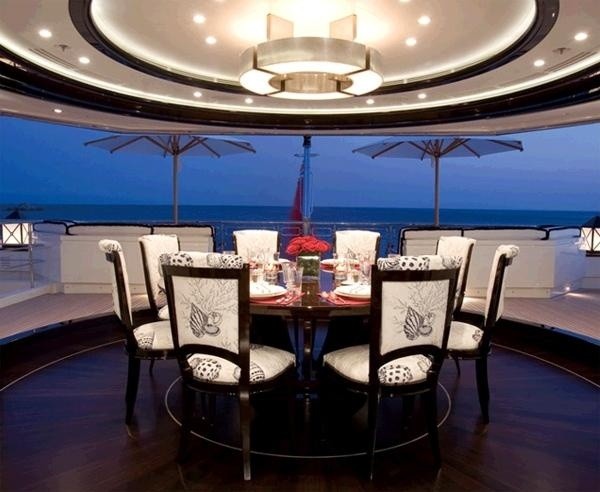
[322,255,463,481]
[156,253,297,481]
[99,228,520,427]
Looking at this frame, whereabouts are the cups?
[319,270,333,292]
[281,262,297,285]
[333,252,371,286]
[287,267,304,292]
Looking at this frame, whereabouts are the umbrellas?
[82,131,257,224]
[351,134,525,227]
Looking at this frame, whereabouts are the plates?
[334,283,371,300]
[250,284,287,299]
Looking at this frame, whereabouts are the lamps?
[238,14,385,100]
[1,222,33,247]
[579,226,600,257]
